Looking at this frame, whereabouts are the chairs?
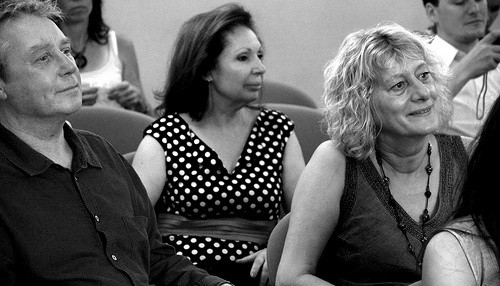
[68,79,331,286]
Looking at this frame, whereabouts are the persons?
[422,94,500,286]
[275,20,468,286]
[0,0,234,286]
[423,0,500,140]
[47,0,147,114]
[132,3,307,286]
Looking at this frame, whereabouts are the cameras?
[479,36,500,64]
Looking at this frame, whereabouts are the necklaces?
[70,40,89,70]
[373,141,433,270]
